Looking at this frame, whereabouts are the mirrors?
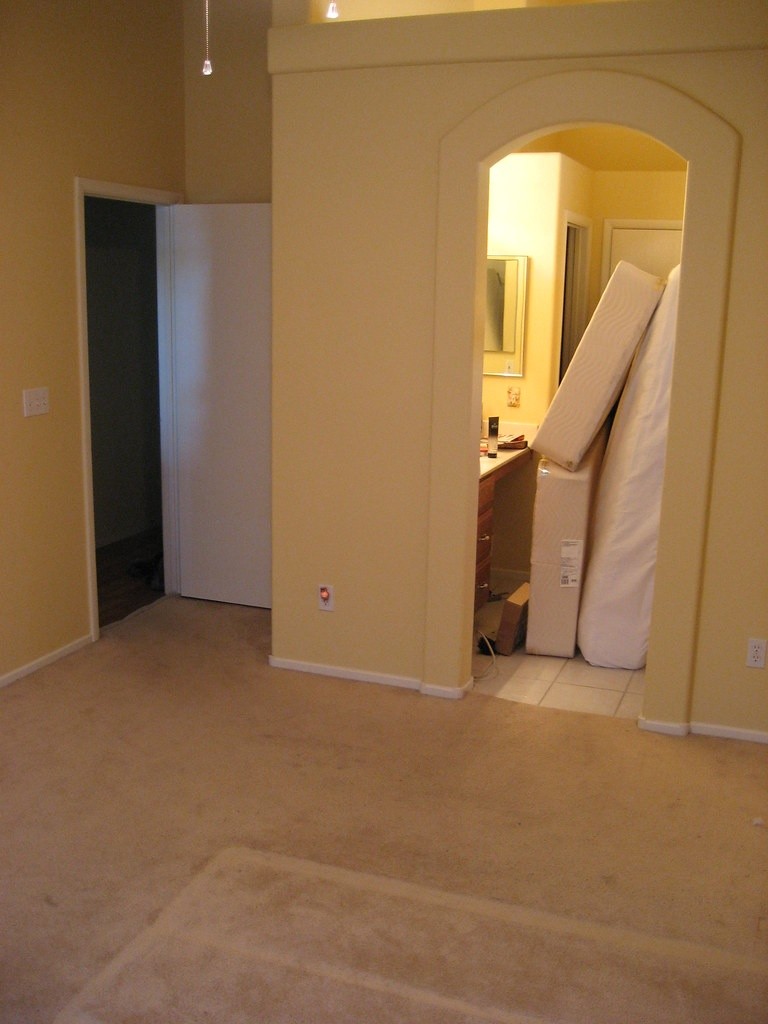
[483,255,529,377]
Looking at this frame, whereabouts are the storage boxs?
[495,580,530,656]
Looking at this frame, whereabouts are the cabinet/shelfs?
[475,474,496,613]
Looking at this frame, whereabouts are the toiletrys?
[488,417,499,458]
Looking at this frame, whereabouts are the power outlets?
[745,637,766,669]
[319,584,335,612]
[507,385,521,408]
